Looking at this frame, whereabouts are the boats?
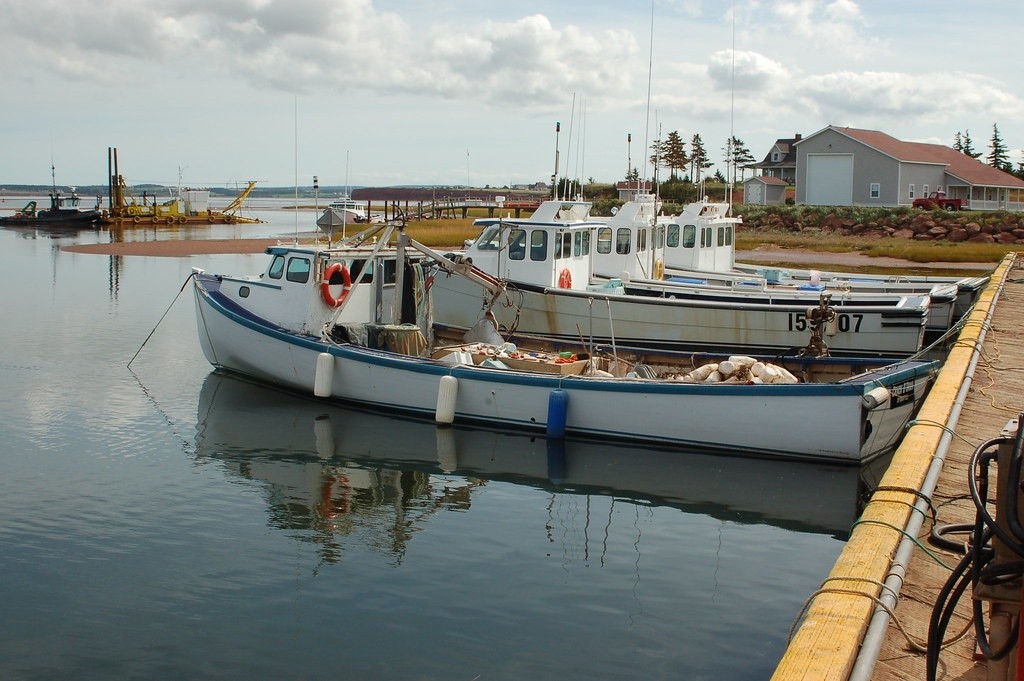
[422,91,993,359]
[188,94,939,469]
[185,370,894,569]
[98,146,270,225]
[326,149,384,224]
[0,158,101,226]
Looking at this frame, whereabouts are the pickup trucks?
[912,191,969,213]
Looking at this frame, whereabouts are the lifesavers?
[151,216,159,223]
[224,215,232,223]
[178,215,187,224]
[655,258,663,280]
[323,263,351,307]
[559,268,572,289]
[134,216,141,223]
[166,216,175,224]
[208,215,215,222]
[115,217,123,226]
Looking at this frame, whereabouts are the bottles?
[675,355,798,384]
